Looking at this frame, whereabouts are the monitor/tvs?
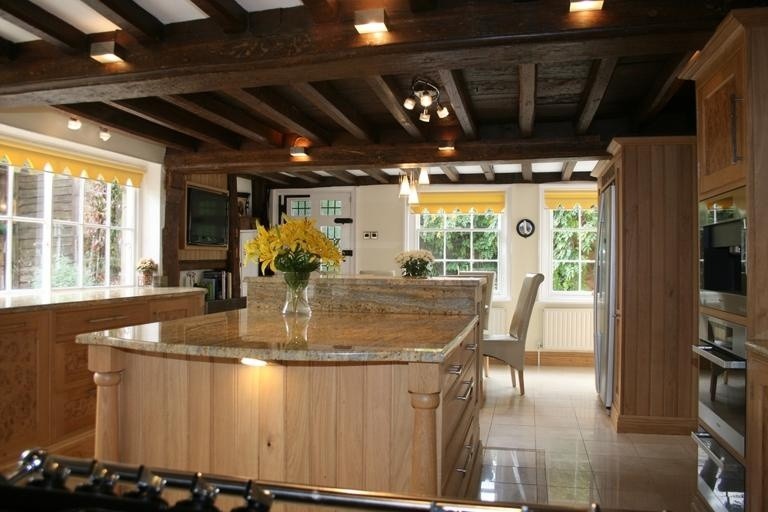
[187,186,227,248]
[703,218,746,297]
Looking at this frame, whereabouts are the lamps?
[399,72,449,127]
[287,146,311,158]
[88,38,130,67]
[66,116,84,130]
[351,7,393,34]
[98,126,112,141]
[394,163,434,209]
[436,140,456,152]
[566,0,605,15]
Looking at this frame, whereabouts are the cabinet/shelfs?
[671,2,768,511]
[1,285,213,482]
[585,130,701,441]
[70,303,487,503]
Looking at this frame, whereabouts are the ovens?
[692,179,750,511]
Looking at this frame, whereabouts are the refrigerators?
[591,183,618,405]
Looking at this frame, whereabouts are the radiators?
[532,298,597,357]
[482,305,506,336]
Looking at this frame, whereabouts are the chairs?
[452,268,496,382]
[476,269,546,398]
[358,266,398,282]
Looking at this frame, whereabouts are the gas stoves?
[0,449,528,511]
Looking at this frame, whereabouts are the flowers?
[239,205,349,317]
[132,256,161,273]
[392,247,438,281]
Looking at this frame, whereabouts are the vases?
[138,268,154,288]
[274,267,313,318]
